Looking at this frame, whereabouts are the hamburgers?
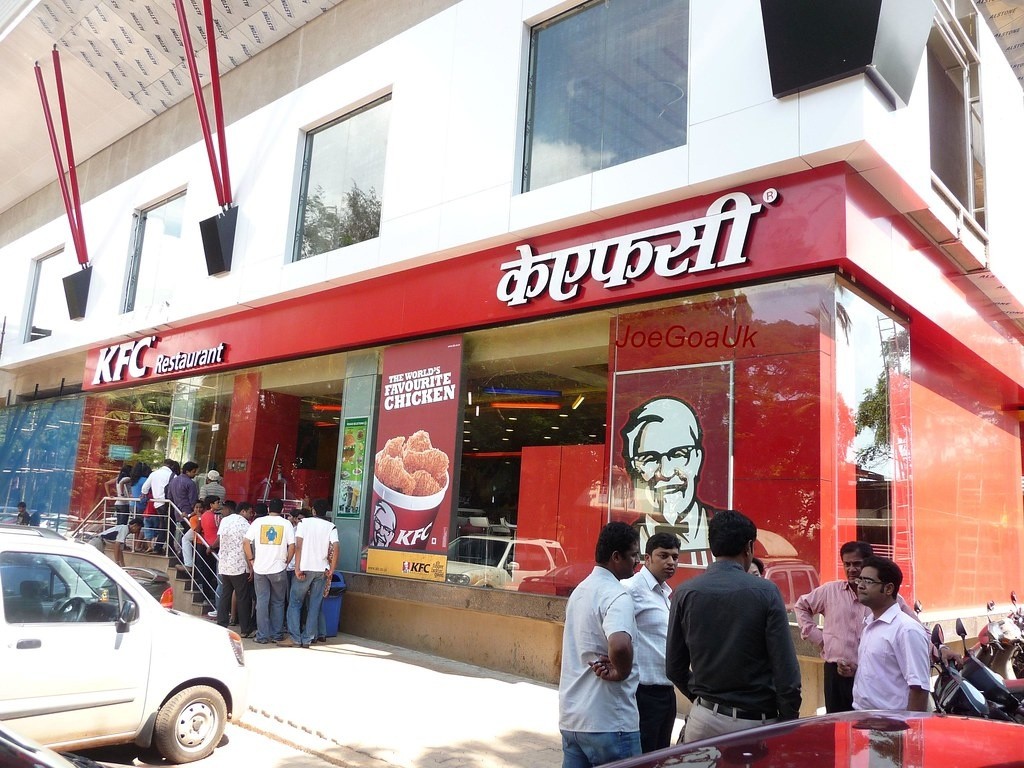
[343,435,356,459]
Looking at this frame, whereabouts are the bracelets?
[187,514,192,519]
[120,494,125,497]
[248,558,254,562]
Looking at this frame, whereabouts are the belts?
[698,697,777,721]
[95,536,106,545]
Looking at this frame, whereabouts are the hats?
[208,470,219,480]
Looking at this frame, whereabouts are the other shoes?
[149,552,159,555]
[252,637,268,643]
[145,548,154,553]
[203,600,215,604]
[197,584,204,590]
[134,547,145,552]
[302,644,309,648]
[175,565,193,573]
[229,619,236,625]
[277,638,301,647]
[240,631,258,638]
[318,637,326,642]
[208,611,231,617]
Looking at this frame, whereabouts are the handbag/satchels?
[164,473,175,504]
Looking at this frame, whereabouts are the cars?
[1,522,245,765]
[0,506,133,545]
[519,561,595,598]
[0,553,174,622]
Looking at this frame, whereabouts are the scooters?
[930,588,1024,725]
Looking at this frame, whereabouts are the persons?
[87,518,145,567]
[15,501,30,526]
[664,509,803,748]
[105,459,238,623]
[618,531,683,752]
[276,499,340,649]
[243,497,296,645]
[557,522,643,768]
[744,557,766,581]
[252,502,268,521]
[286,506,310,600]
[792,541,966,716]
[851,557,935,712]
[216,502,261,639]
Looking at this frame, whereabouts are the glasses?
[298,518,303,521]
[855,578,884,585]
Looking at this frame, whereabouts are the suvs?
[759,557,821,623]
[445,535,567,589]
